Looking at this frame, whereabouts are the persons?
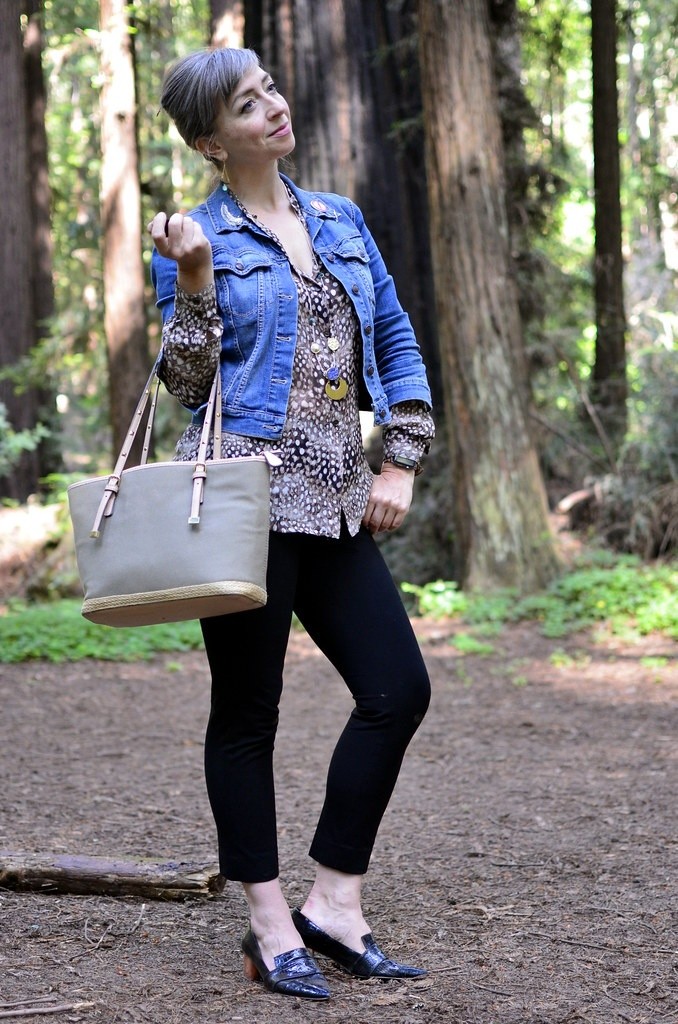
[145,47,436,1001]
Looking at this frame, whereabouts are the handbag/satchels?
[67,340,283,627]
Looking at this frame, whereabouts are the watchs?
[383,456,417,471]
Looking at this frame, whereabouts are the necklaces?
[226,181,347,400]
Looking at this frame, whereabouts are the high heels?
[241,928,330,1000]
[291,907,428,980]
